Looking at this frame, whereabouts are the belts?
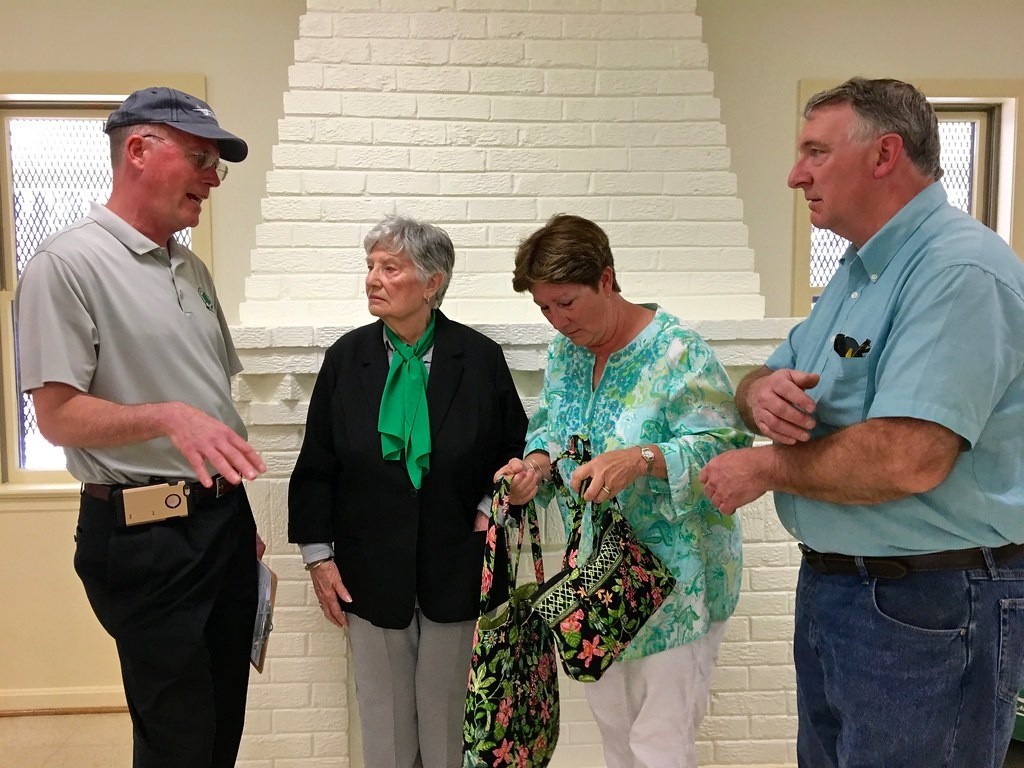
[85,472,241,501]
[798,543,1024,579]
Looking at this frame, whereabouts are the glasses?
[143,135,228,181]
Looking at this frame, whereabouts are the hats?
[104,86,247,163]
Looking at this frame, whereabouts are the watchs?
[635,443,656,477]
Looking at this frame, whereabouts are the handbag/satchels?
[526,508,675,681]
[461,474,560,768]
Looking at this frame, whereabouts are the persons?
[286,214,530,767]
[493,210,756,768]
[698,77,1024,766]
[13,87,269,768]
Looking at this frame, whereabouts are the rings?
[318,601,323,607]
[601,485,612,494]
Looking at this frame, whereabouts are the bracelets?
[304,557,332,571]
[523,458,542,476]
